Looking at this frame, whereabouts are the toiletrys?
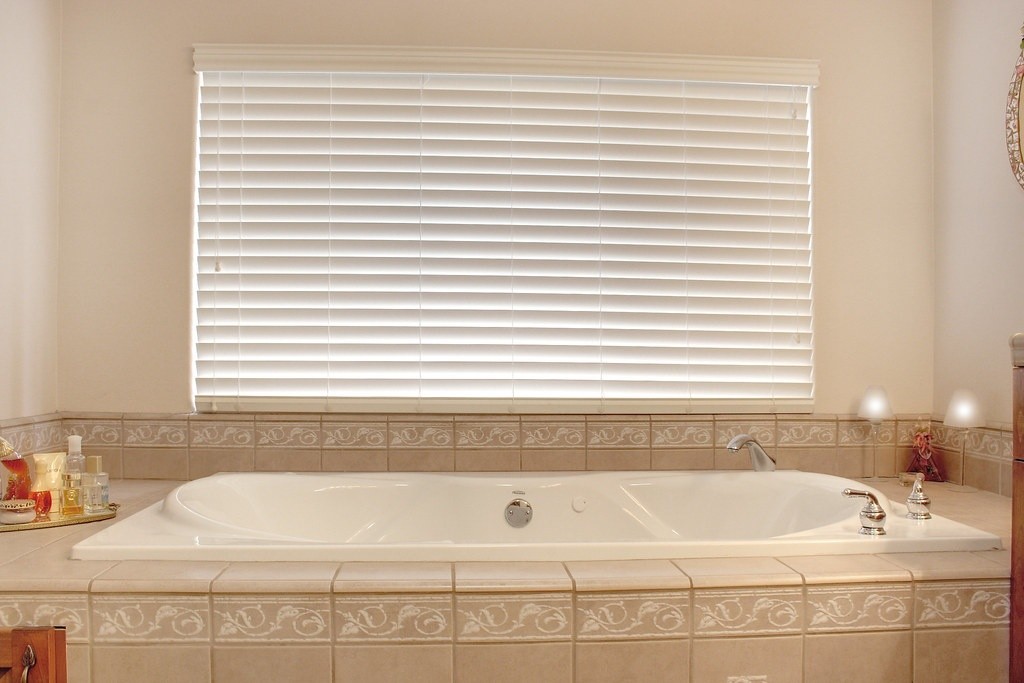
[0,435,31,508]
[66,435,87,480]
[81,454,113,515]
[32,451,65,512]
[59,472,85,517]
[28,459,53,518]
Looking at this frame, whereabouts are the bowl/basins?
[0,499,37,524]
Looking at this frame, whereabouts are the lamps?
[856,385,892,483]
[942,388,983,494]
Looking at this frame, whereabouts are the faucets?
[727,434,779,473]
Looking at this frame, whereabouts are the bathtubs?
[0,453,1011,683]
